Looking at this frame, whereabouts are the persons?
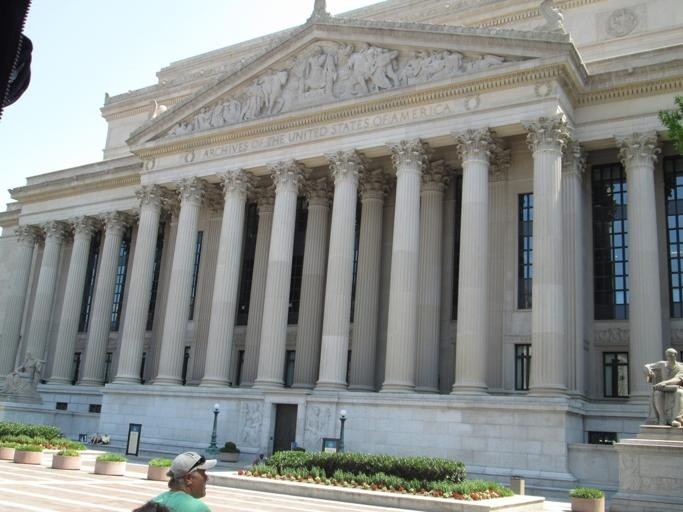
[91,432,101,444]
[100,433,109,445]
[642,347,683,428]
[238,400,263,447]
[136,450,218,511]
[305,405,331,449]
[164,39,504,132]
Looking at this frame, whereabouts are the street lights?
[334,410,346,454]
[206,404,222,453]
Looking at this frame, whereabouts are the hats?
[170,452,218,480]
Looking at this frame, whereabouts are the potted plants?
[569,487,605,511]
[219,441,240,462]
[0,441,21,460]
[147,457,173,481]
[51,449,82,470]
[95,454,127,475]
[13,443,43,465]
